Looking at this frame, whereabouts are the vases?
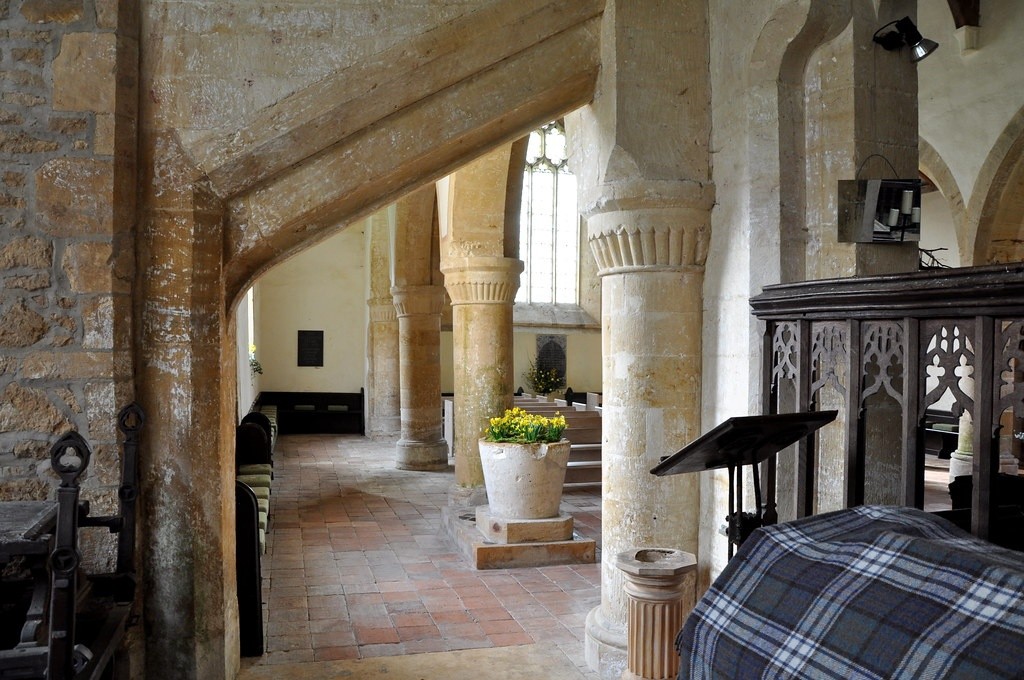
[478,437,572,519]
[547,393,554,402]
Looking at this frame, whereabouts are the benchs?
[235,391,278,658]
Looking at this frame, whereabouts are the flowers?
[485,404,569,444]
[523,356,567,394]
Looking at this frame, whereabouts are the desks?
[0,401,146,680]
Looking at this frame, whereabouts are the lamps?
[872,16,939,64]
